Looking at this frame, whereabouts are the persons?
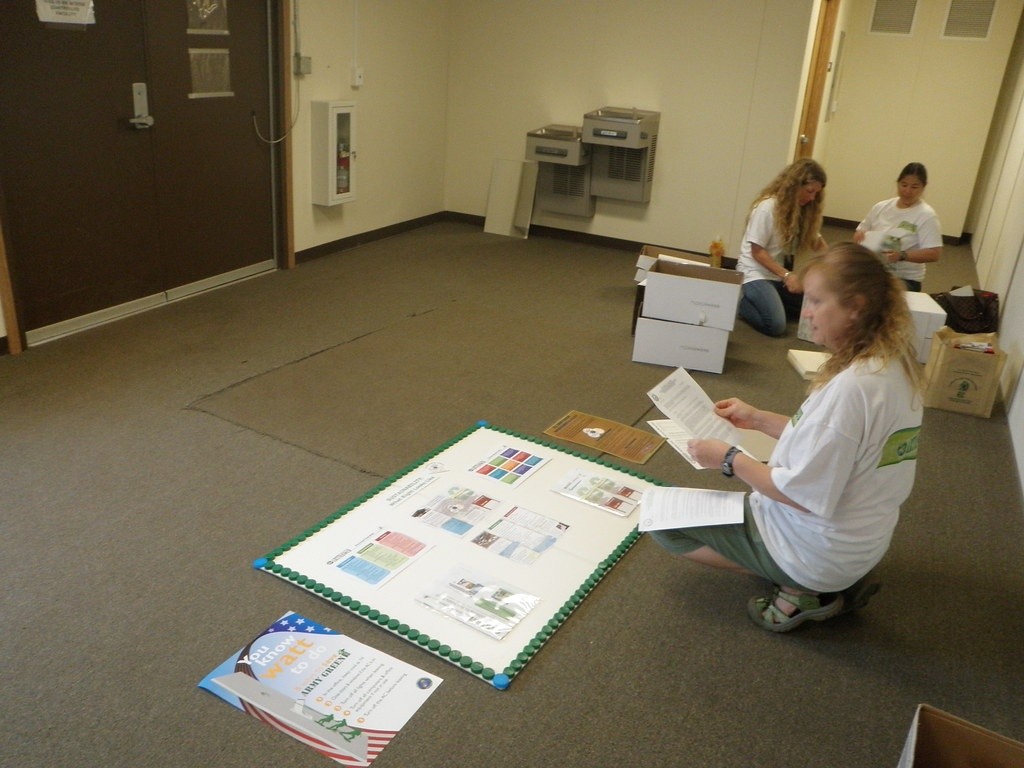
[736,159,828,339]
[650,243,924,632]
[853,162,943,291]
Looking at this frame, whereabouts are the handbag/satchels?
[923,326,1007,418]
[930,285,999,334]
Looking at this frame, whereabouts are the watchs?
[721,447,743,477]
[900,250,908,262]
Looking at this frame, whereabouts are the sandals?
[747,569,844,632]
[841,570,886,624]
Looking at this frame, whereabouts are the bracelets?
[784,271,793,282]
